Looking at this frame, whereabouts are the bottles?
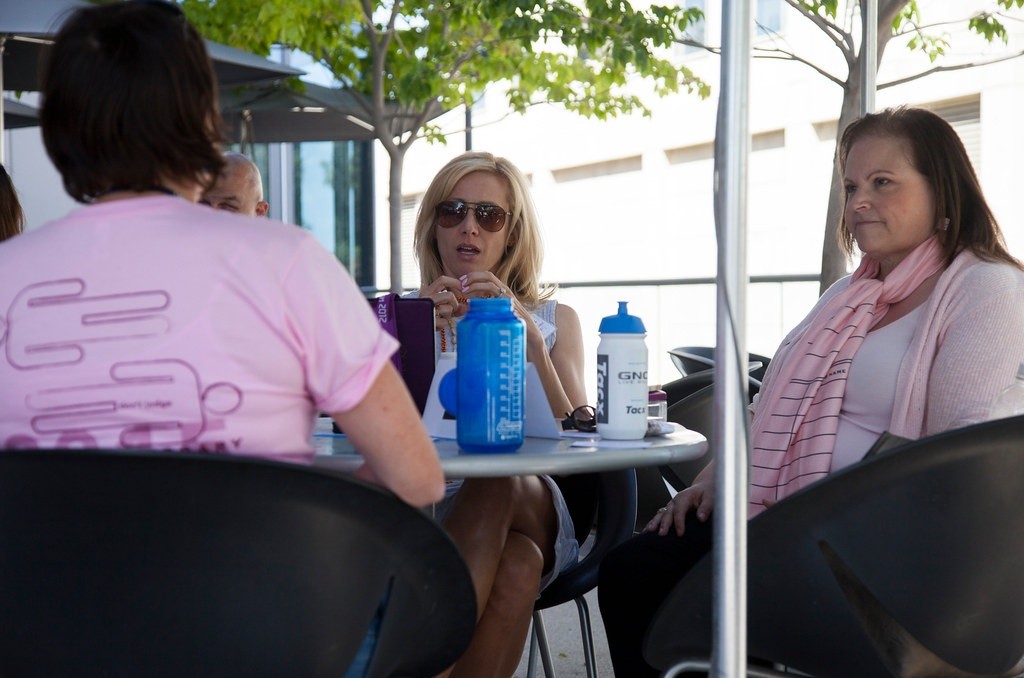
[438,297,524,451]
[597,300,645,440]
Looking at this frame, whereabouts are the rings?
[498,287,507,298]
[659,508,669,513]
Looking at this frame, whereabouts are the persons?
[198,154,268,222]
[0,0,445,508]
[0,166,26,245]
[395,151,602,677]
[596,106,1023,678]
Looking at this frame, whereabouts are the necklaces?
[434,290,493,352]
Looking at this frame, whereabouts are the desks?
[302,415,711,507]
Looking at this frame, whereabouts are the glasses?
[434,199,513,233]
[561,405,595,433]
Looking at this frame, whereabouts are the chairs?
[667,341,776,395]
[490,465,641,678]
[645,413,1024,678]
[0,446,481,678]
[646,361,763,503]
